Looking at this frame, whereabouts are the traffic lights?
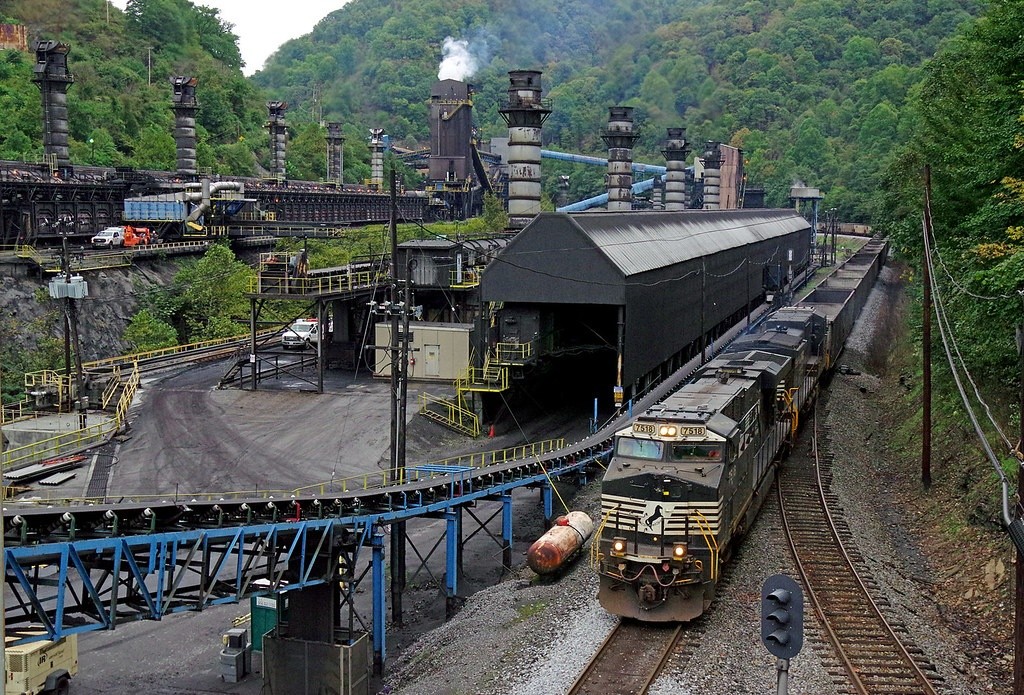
[765,589,790,648]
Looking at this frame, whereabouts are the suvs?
[282,322,324,350]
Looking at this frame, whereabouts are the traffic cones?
[488,424,495,438]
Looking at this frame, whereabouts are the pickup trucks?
[91,227,126,249]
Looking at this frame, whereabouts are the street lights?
[90,138,95,165]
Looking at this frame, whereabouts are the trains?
[591,222,891,622]
